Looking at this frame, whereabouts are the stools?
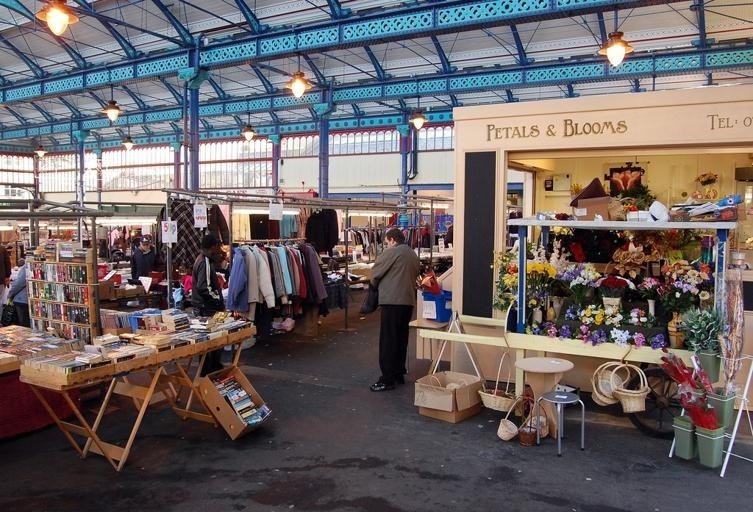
[537,392,584,457]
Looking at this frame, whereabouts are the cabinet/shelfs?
[0,226,453,471]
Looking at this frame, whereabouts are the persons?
[369,229,420,392]
[113,231,225,377]
[7,258,31,329]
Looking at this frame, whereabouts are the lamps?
[121,136,136,151]
[34,145,48,157]
[597,30,635,67]
[409,111,429,129]
[33,0,79,36]
[101,100,124,121]
[286,71,313,98]
[240,126,255,141]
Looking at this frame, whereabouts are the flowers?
[491,171,720,350]
[660,347,717,425]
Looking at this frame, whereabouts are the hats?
[140,237,149,242]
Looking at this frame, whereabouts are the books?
[23,243,91,344]
[1,324,79,366]
[212,374,270,425]
[26,308,251,374]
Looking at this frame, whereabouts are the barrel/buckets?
[423,291,452,323]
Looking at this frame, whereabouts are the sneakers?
[370,375,405,392]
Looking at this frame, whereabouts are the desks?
[515,356,573,438]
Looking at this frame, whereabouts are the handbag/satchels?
[360,283,378,314]
[2,297,15,326]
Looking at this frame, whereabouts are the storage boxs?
[414,371,484,424]
